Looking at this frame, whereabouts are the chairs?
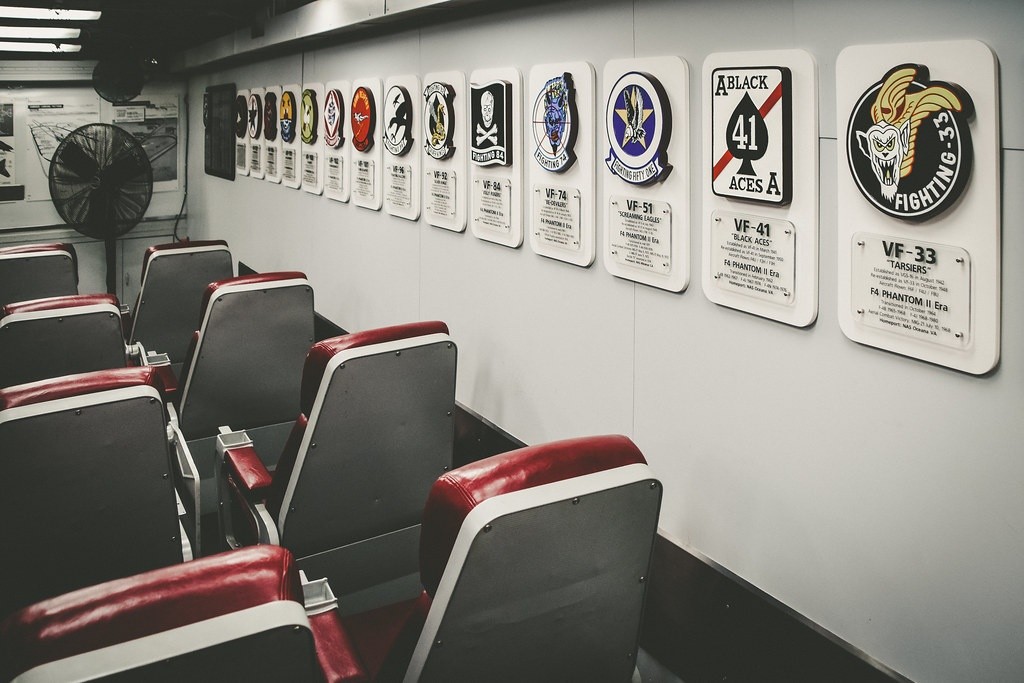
[1,240,663,683]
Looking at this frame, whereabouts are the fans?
[93,57,144,103]
[49,123,153,295]
